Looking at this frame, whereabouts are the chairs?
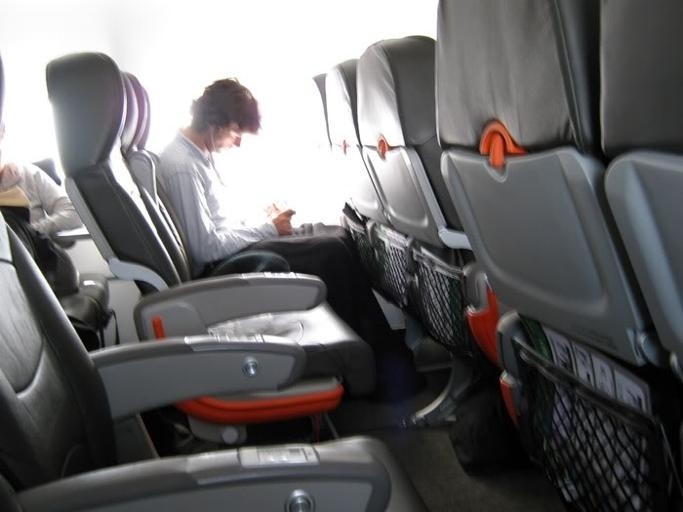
[0,204,122,354]
[1,214,392,511]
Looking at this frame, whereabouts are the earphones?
[211,124,215,128]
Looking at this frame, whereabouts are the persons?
[0,161,83,298]
[156,79,427,403]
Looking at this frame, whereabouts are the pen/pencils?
[271,203,282,214]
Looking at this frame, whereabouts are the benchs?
[310,33,513,482]
[431,0,681,511]
[45,51,379,447]
